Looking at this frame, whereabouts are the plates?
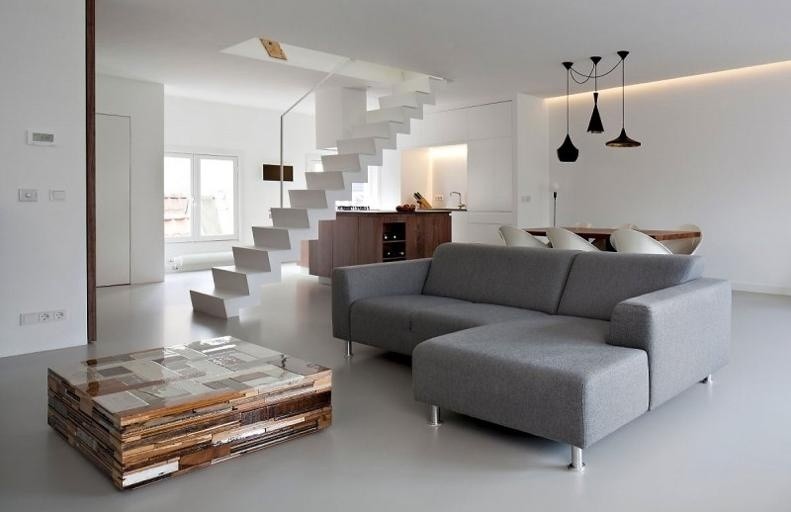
[396,207,416,212]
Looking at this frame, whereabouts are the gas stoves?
[336,205,372,211]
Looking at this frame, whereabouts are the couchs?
[332,242,731,471]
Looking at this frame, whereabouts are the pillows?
[421,242,704,321]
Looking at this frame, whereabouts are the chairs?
[498,222,704,255]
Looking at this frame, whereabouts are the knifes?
[409,192,422,201]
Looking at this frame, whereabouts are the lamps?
[557,51,641,162]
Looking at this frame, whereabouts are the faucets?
[450,192,461,205]
